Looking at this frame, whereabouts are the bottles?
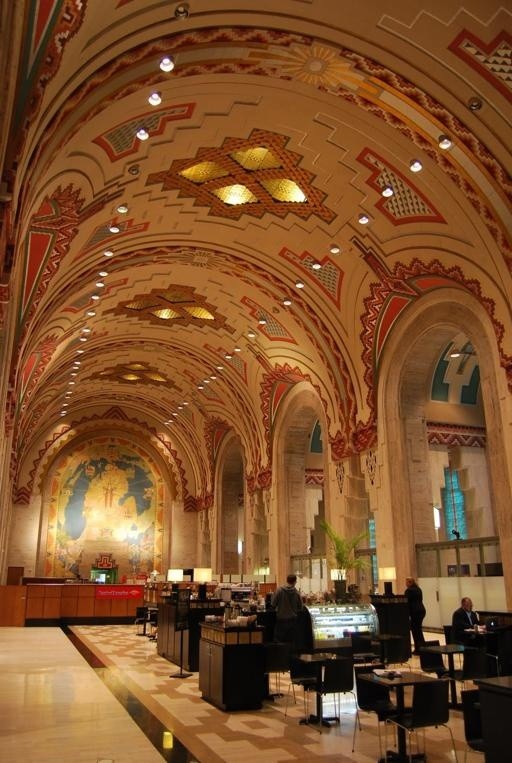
[222,602,248,622]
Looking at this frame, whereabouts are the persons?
[403,576,426,655]
[451,596,489,678]
[271,574,304,649]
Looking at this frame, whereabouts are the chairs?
[135,603,158,641]
[265,622,504,763]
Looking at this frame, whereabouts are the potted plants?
[316,516,377,597]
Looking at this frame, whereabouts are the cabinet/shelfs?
[159,598,265,712]
